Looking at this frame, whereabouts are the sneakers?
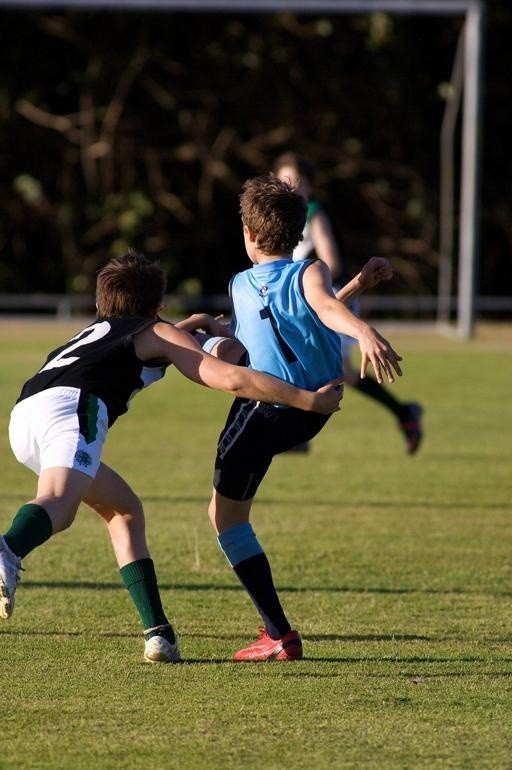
[142,637,182,664]
[399,402,421,453]
[234,625,302,660]
[0,534,24,619]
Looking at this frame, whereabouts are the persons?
[0,252,346,663]
[159,174,404,663]
[268,151,424,459]
